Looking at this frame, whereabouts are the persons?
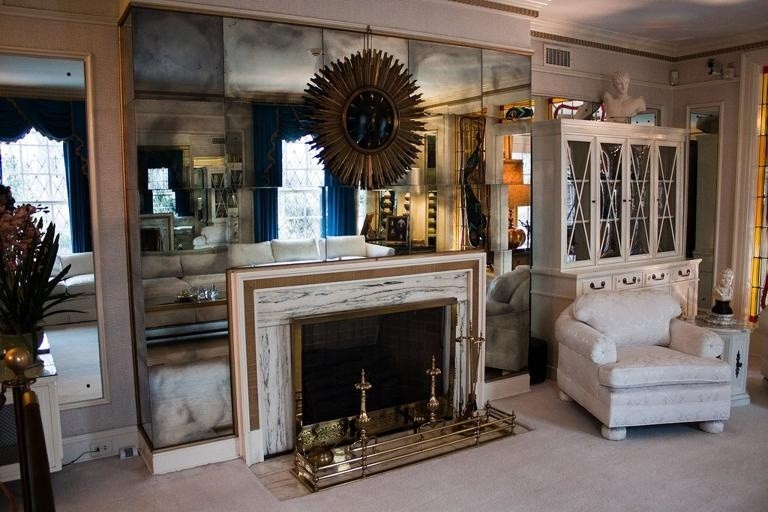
[395,218,406,242]
[603,68,647,118]
[713,266,736,302]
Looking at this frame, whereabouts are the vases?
[0,328,44,350]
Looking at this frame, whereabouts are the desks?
[32,332,51,353]
[690,318,756,409]
[0,330,61,512]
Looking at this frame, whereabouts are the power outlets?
[91,441,113,457]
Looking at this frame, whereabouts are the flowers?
[0,185,98,330]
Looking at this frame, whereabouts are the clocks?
[298,43,428,193]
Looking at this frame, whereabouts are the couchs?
[37,250,97,326]
[139,237,396,329]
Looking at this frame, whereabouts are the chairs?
[552,289,730,443]
[485,263,531,374]
[192,226,233,248]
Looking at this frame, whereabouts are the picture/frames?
[385,213,409,245]
[140,212,175,253]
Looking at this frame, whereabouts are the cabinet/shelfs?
[642,260,699,333]
[531,268,643,346]
[530,118,701,272]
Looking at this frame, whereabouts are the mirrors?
[1,43,112,414]
[115,0,534,454]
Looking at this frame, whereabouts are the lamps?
[504,184,533,249]
